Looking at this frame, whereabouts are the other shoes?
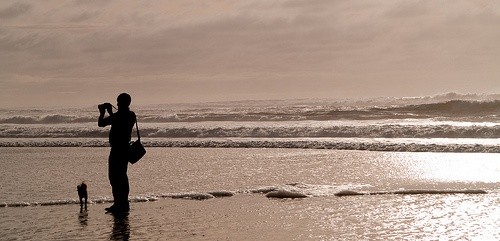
[104,204,130,211]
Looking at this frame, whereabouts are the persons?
[97,93,136,213]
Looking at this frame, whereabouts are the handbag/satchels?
[127,140,147,165]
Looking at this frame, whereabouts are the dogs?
[76,181,88,205]
